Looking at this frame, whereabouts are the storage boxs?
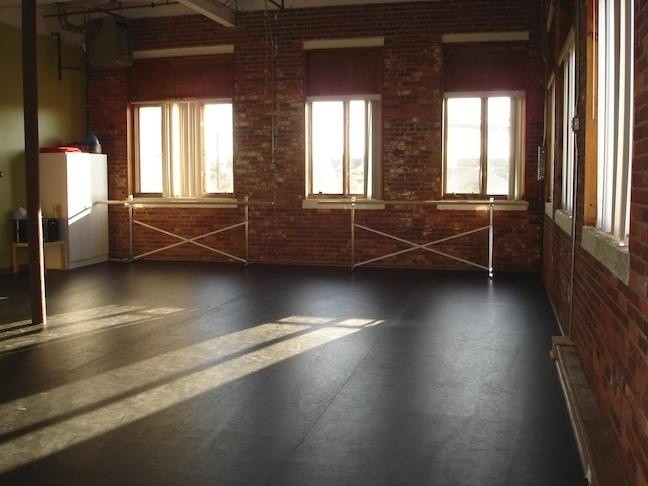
[14,217,59,242]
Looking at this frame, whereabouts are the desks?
[12,239,65,273]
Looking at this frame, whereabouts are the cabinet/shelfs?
[39,151,109,269]
